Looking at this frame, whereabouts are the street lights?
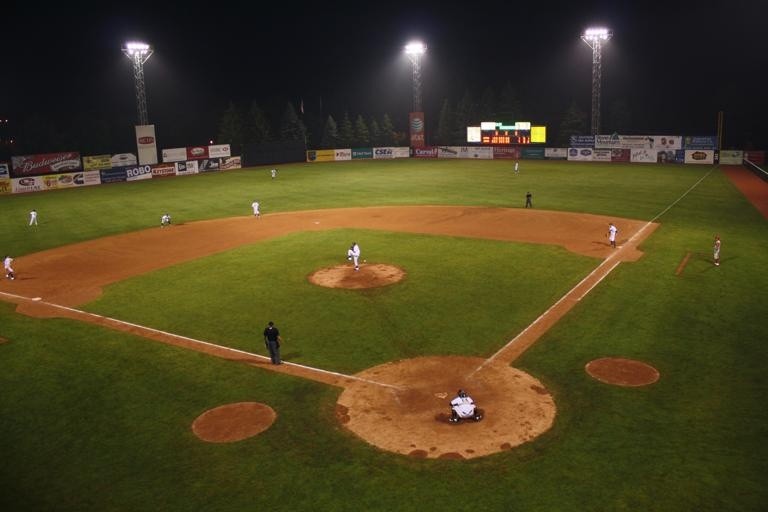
[580,27,614,134]
[120,40,154,125]
[405,41,428,113]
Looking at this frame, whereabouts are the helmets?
[458,390,466,395]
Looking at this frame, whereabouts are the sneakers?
[476,414,482,420]
[449,418,457,422]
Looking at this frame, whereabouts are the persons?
[514,159,519,175]
[160,214,171,228]
[448,389,481,423]
[271,167,276,178]
[606,223,618,246]
[347,242,360,271]
[712,235,721,266]
[29,209,38,225]
[251,200,260,218]
[263,321,284,365]
[4,255,15,280]
[525,192,533,208]
